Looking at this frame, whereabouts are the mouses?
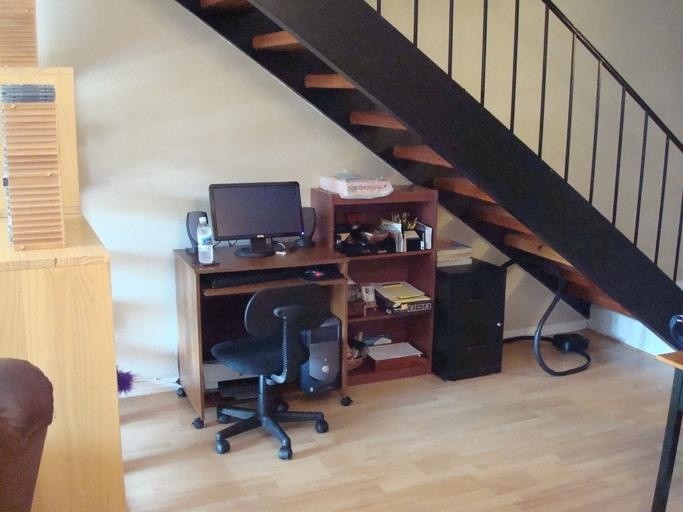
[304,266,326,279]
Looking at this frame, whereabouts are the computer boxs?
[294,312,340,395]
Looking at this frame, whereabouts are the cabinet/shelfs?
[310,184,440,386]
[433,255,508,380]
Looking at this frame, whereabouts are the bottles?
[197,217,214,265]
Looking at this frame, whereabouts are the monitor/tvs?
[209,181,304,258]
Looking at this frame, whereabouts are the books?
[435,239,474,269]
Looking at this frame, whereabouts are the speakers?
[294,207,316,248]
[186,211,208,256]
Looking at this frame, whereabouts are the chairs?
[214,283,329,459]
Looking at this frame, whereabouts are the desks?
[651,350,682,512]
[173,238,354,429]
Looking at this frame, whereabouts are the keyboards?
[207,268,293,289]
